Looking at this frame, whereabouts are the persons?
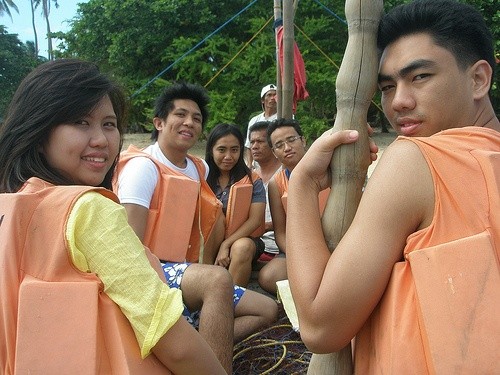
[248,121,284,262]
[204,123,266,289]
[286,0,500,375]
[0,58,229,375]
[257,118,331,297]
[244,84,277,171]
[111,85,277,374]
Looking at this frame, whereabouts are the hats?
[261,84,277,112]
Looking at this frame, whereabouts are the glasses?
[272,135,302,151]
